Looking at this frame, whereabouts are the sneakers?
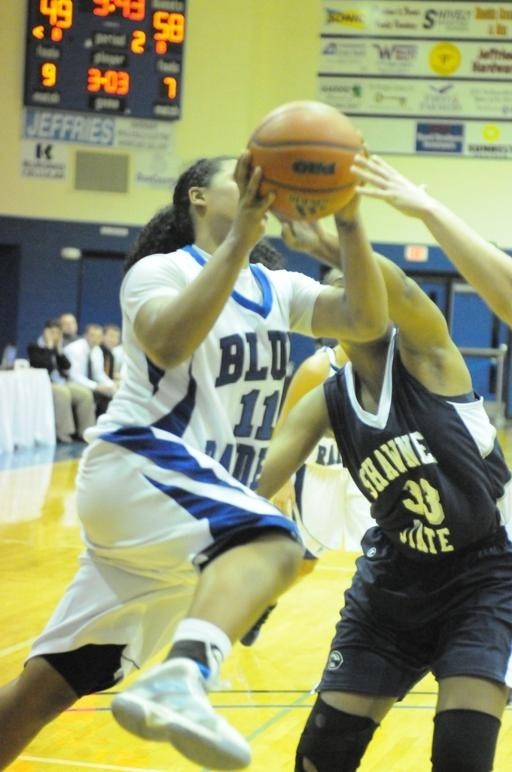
[111,660,249,771]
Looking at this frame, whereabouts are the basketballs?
[245,99,368,225]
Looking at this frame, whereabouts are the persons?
[255,219,511,772]
[27,311,124,444]
[241,340,375,646]
[348,152,511,325]
[0,150,388,772]
